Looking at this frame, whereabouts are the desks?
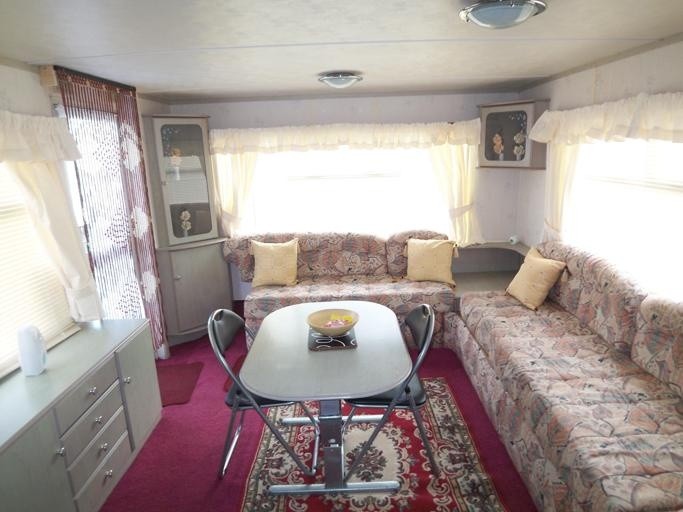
[238,300,412,495]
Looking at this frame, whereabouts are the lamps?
[318,72,363,90]
[457,0,547,28]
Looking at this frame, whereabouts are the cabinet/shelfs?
[0,319,162,512]
[476,97,551,170]
[141,113,232,349]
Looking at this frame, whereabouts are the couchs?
[445,241,683,512]
[220,230,454,350]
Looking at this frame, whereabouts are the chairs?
[340,302,442,483]
[203,306,322,481]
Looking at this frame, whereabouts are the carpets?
[234,376,512,512]
[156,362,204,407]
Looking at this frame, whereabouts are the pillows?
[250,238,299,288]
[407,239,456,287]
[505,247,567,311]
